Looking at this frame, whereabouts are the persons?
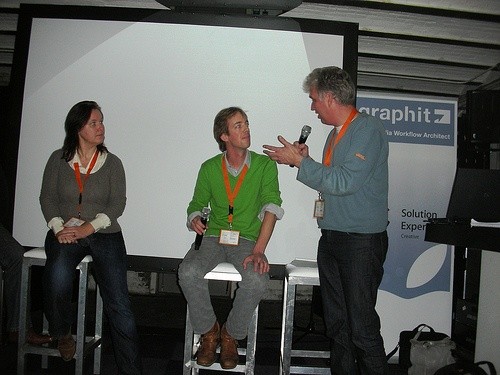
[39,101,141,375]
[177,107,284,369]
[263,66,392,375]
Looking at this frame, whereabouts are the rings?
[73,233,77,237]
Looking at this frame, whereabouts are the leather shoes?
[219,320,240,369]
[55,326,77,363]
[7,327,51,345]
[196,321,221,367]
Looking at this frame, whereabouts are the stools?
[279,259,332,374]
[183,262,259,375]
[16,248,103,375]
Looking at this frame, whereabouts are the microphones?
[194,207,210,250]
[290,124,312,168]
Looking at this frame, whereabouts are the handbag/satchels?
[385,323,458,375]
